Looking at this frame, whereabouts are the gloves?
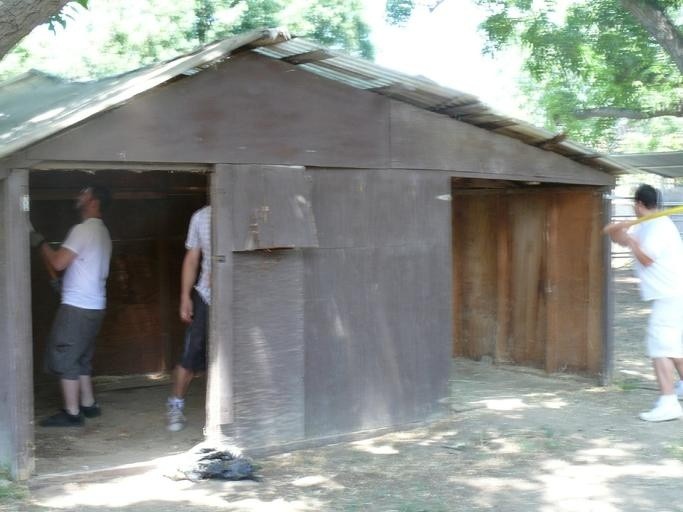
[30,231,45,248]
[47,277,62,295]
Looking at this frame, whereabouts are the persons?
[163,205,211,432]
[29,182,112,428]
[608,184,683,422]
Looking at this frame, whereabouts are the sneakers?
[638,380,683,422]
[165,395,187,431]
[38,402,101,427]
